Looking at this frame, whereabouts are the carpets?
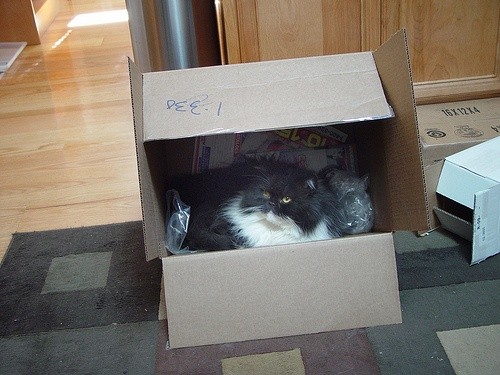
[0,221,500,375]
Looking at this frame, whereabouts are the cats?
[163,159,348,251]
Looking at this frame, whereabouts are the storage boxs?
[127,28,431,350]
[415,97,500,165]
[417,135,500,266]
